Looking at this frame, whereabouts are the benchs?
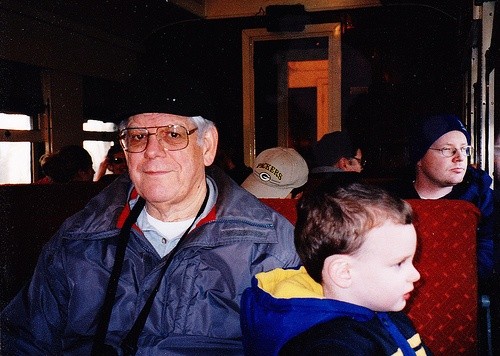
[0,182,110,312]
[254,198,481,356]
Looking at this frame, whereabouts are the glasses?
[427,143,474,157]
[353,156,368,170]
[108,157,125,164]
[119,125,198,153]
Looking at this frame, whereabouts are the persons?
[95,144,129,182]
[377,115,500,356]
[0,82,302,356]
[298,131,382,191]
[30,145,95,184]
[239,180,438,356]
[240,146,322,225]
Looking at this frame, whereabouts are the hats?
[408,109,471,166]
[310,131,362,167]
[241,147,309,199]
[108,64,216,124]
